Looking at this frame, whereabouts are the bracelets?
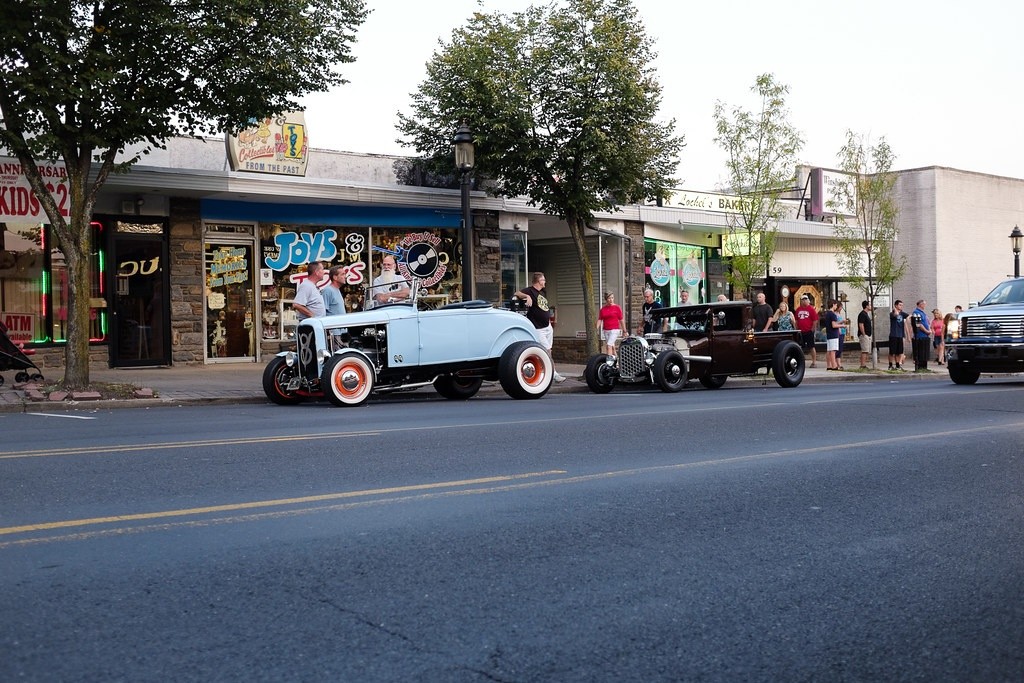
[623,332,627,333]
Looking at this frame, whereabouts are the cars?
[943,277,1024,385]
[262,277,555,408]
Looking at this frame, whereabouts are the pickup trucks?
[585,301,806,395]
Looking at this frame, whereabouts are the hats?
[605,291,614,300]
[800,295,808,300]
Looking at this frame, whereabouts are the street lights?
[1008,224,1024,279]
[451,119,477,302]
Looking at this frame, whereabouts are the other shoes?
[938,361,944,365]
[810,363,817,368]
[838,365,844,370]
[555,374,566,382]
[926,368,931,371]
[896,365,902,370]
[827,366,838,370]
[860,365,868,368]
[888,366,894,370]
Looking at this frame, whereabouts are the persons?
[955,306,962,313]
[637,289,668,337]
[292,261,326,323]
[777,302,796,331]
[596,290,627,355]
[826,299,849,370]
[513,272,566,384]
[794,295,819,368]
[930,309,945,365]
[857,300,876,369]
[752,293,773,332]
[718,294,727,302]
[321,265,348,352]
[676,289,692,307]
[372,256,410,307]
[888,299,932,370]
